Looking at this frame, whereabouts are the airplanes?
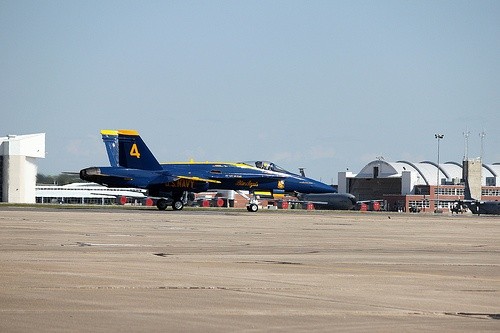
[286,192,384,210]
[424,176,500,215]
[61,129,338,212]
